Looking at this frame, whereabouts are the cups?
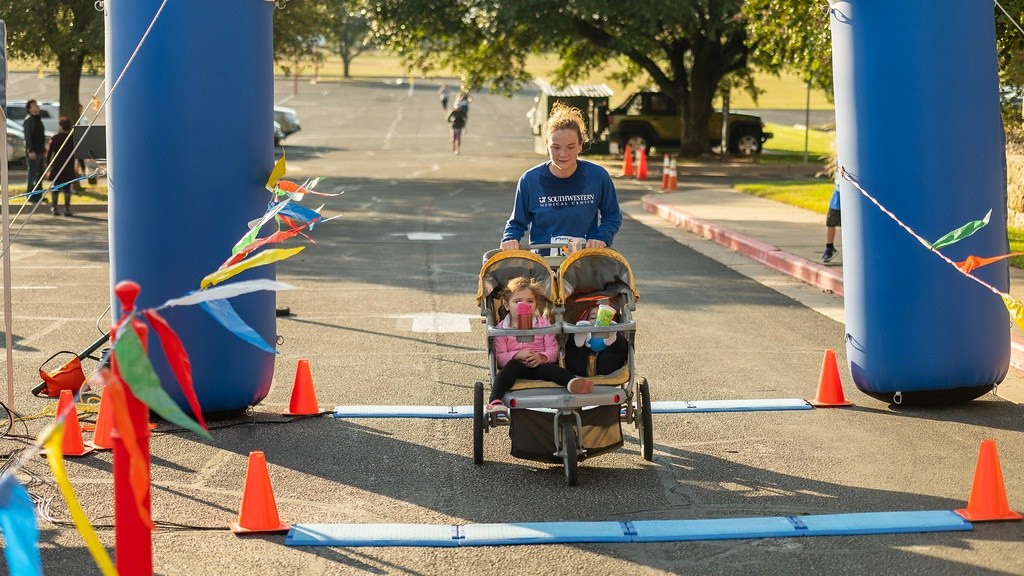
[594,305,616,326]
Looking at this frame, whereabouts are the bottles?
[516,301,532,330]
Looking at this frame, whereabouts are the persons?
[499,99,622,251]
[565,301,629,377]
[486,276,594,413]
[78,104,86,176]
[22,100,47,202]
[440,83,449,109]
[821,159,841,262]
[46,117,75,217]
[448,91,468,154]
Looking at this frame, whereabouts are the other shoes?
[64,210,72,216]
[52,209,60,216]
[486,400,509,414]
[567,378,593,394]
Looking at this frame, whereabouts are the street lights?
[84,383,114,450]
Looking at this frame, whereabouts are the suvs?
[608,90,774,159]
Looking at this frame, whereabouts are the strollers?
[473,238,654,486]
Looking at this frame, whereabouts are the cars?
[273,107,302,147]
[7,99,90,167]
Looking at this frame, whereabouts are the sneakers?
[820,248,839,262]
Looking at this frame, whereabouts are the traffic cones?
[634,147,652,180]
[615,144,637,179]
[38,389,95,458]
[952,439,1024,522]
[661,155,685,193]
[659,153,671,191]
[281,360,337,418]
[803,349,853,407]
[229,449,291,534]
